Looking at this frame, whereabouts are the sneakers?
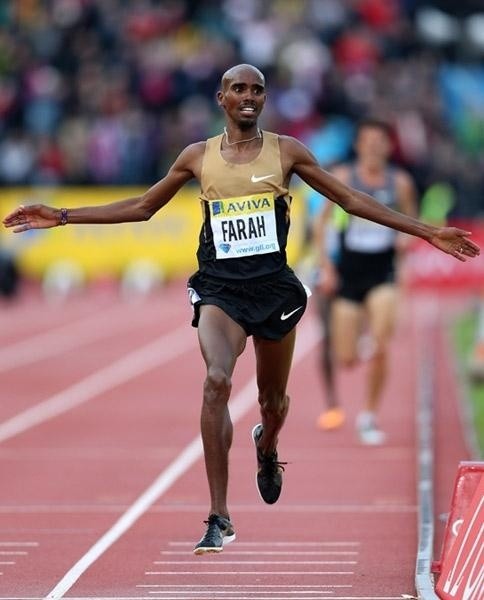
[196,514,235,553]
[252,424,284,504]
[356,413,385,446]
[318,407,343,431]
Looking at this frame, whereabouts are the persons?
[4,63,481,553]
[1,2,483,448]
